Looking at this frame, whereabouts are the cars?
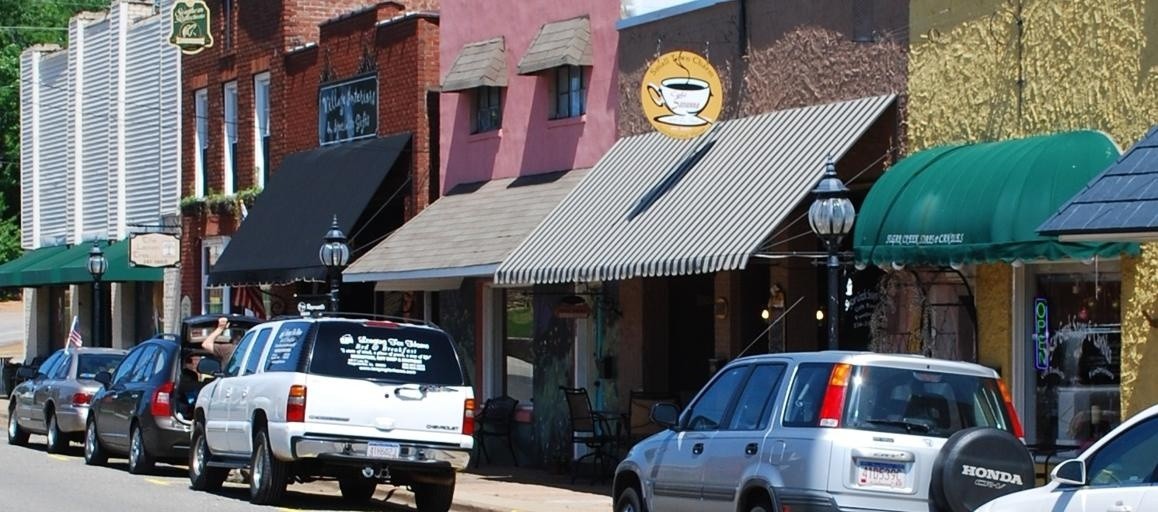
[504,336,535,407]
[971,399,1158,512]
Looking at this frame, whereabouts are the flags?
[68,317,83,347]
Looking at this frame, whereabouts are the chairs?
[630,389,681,444]
[470,395,523,472]
[560,381,632,482]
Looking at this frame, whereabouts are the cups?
[648,76,711,117]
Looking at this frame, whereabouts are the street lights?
[321,213,350,314]
[83,241,108,346]
[808,153,857,350]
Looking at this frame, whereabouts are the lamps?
[761,283,780,323]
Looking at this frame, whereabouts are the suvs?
[1038,321,1120,451]
[608,348,1034,512]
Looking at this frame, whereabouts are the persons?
[200,316,245,482]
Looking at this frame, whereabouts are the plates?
[653,115,708,126]
